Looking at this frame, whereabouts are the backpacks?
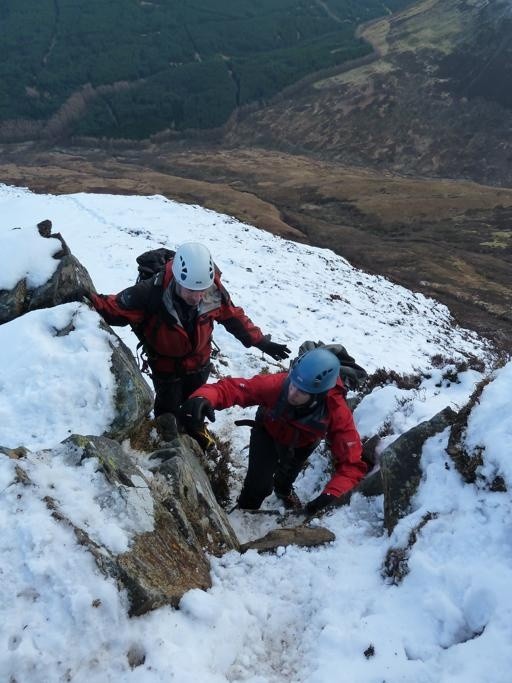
[136,247,223,371]
[234,340,364,458]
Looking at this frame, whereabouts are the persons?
[179,348,369,514]
[88,242,291,446]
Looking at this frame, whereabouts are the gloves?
[256,333,291,361]
[306,493,332,516]
[177,395,215,431]
[62,287,93,303]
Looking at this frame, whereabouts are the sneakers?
[280,488,302,510]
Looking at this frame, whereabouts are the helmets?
[171,242,215,291]
[290,347,340,394]
